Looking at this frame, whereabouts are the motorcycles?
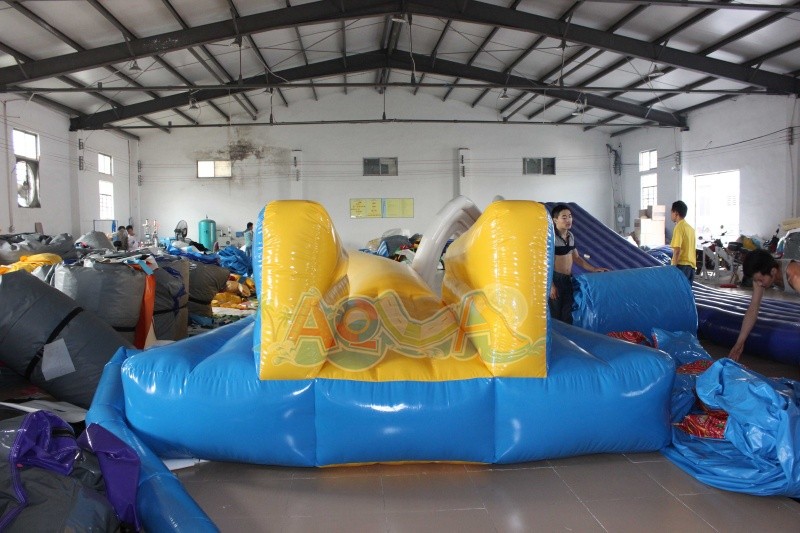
[696,224,733,275]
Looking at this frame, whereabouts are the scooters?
[728,226,780,274]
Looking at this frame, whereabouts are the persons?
[244,223,254,257]
[550,206,612,324]
[670,201,696,289]
[728,248,800,363]
[112,226,127,250]
[126,225,138,250]
